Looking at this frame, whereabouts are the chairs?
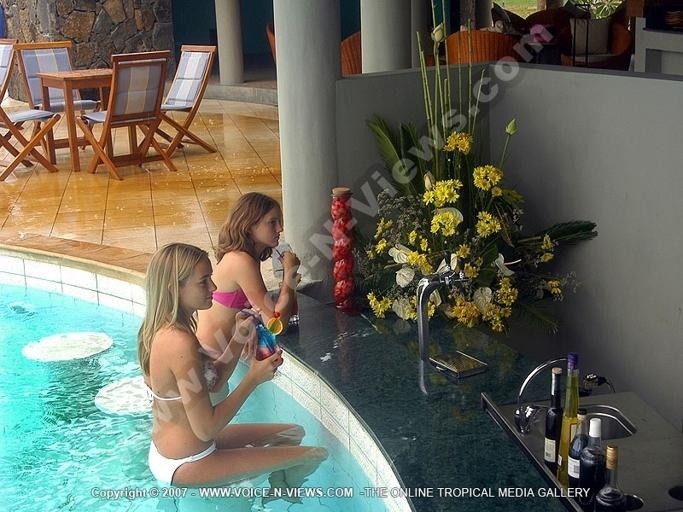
[340,31,362,74]
[446,31,524,66]
[266,22,275,65]
[0,39,217,182]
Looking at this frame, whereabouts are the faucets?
[515,356,568,432]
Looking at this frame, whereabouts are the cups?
[279,283,299,326]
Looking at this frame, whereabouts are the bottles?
[542,353,628,512]
[256,321,276,360]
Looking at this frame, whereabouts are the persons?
[188,187,302,406]
[138,240,329,510]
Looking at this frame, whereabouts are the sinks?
[577,402,635,442]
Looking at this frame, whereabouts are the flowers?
[350,0,599,332]
